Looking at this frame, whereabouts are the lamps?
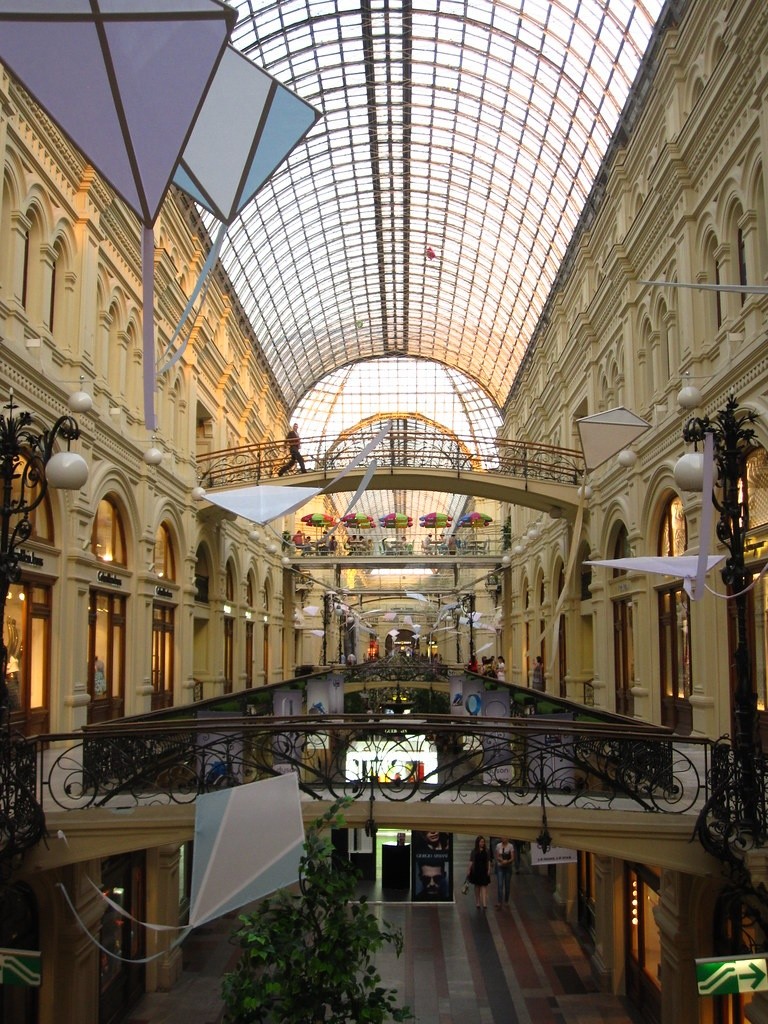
[618,403,668,466]
[249,531,291,565]
[110,407,163,466]
[163,451,205,501]
[671,330,743,409]
[25,337,93,413]
[550,505,562,519]
[502,512,549,564]
[578,474,598,500]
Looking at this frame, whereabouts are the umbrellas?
[300,511,494,548]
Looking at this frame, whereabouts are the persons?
[340,652,346,664]
[469,653,546,693]
[348,652,357,665]
[363,650,444,665]
[278,422,308,476]
[495,834,514,909]
[507,838,523,873]
[418,830,449,850]
[465,835,492,908]
[416,859,450,900]
[279,527,460,559]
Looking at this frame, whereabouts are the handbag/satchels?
[461,880,469,895]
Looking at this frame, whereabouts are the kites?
[56,769,311,964]
[582,431,768,601]
[523,403,654,681]
[200,420,393,549]
[0,0,324,433]
[301,587,498,656]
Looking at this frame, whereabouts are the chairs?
[290,540,415,555]
[421,537,490,555]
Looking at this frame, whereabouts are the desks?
[310,542,323,556]
[470,541,483,555]
[350,541,362,555]
[430,541,442,555]
[391,541,403,555]
